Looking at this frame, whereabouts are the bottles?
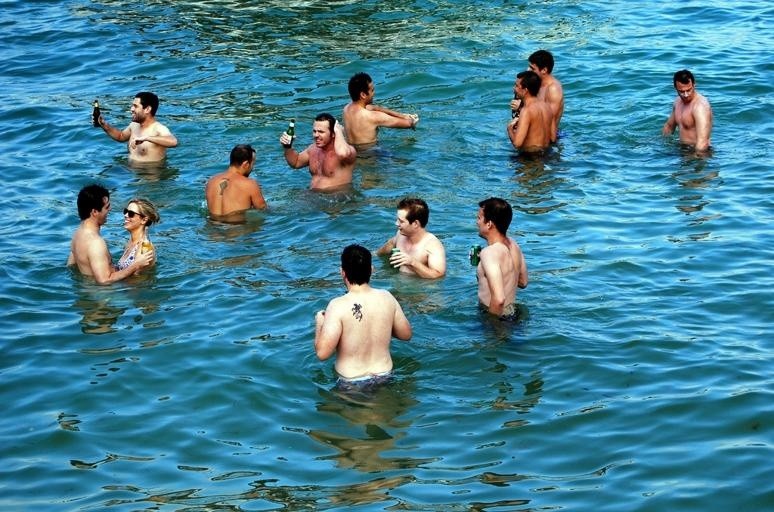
[92,99,102,128]
[140,226,153,256]
[282,119,295,149]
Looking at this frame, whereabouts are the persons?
[89,91,178,164]
[662,70,712,154]
[312,243,412,392]
[467,197,529,325]
[505,71,557,153]
[340,73,419,145]
[113,196,159,275]
[508,48,565,130]
[203,144,269,224]
[63,184,154,285]
[369,197,446,281]
[278,112,357,192]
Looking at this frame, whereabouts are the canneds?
[392,249,401,264]
[471,245,481,266]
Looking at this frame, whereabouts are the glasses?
[123,208,146,218]
